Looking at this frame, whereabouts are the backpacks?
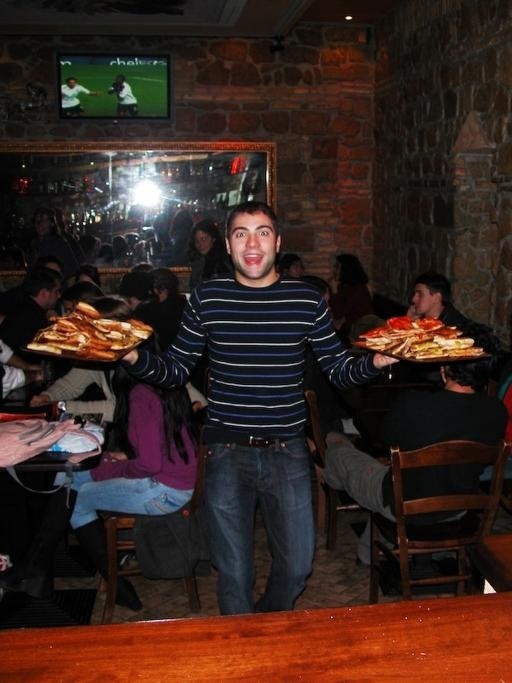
[0,417,102,494]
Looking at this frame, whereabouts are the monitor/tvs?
[57,52,176,126]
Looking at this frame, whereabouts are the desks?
[2,592,512,682]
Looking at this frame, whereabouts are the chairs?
[101,400,203,622]
[306,379,511,604]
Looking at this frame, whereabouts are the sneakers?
[326,431,355,450]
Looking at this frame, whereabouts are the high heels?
[114,576,143,612]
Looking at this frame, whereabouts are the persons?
[61,78,101,115]
[188,219,234,292]
[121,201,400,616]
[108,74,138,117]
[0,200,194,268]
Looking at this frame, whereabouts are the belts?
[198,423,303,448]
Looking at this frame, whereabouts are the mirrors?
[0,138,280,277]
